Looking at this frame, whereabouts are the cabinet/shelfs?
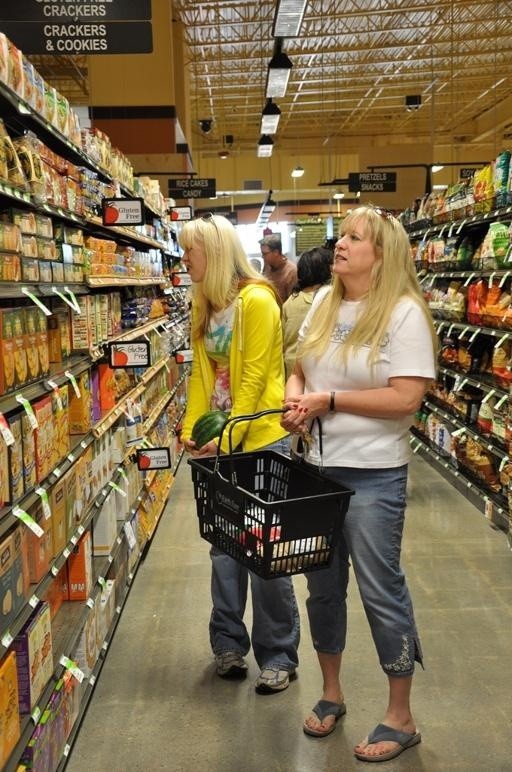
[403,151,512,549]
[0,76,195,772]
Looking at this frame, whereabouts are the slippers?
[354,724,421,762]
[303,700,346,737]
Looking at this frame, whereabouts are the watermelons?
[191,411,234,453]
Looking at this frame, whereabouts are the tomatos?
[171,210,178,221]
[111,346,129,367]
[175,351,185,364]
[172,273,181,287]
[137,452,152,469]
[102,200,119,226]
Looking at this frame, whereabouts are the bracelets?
[328,391,337,415]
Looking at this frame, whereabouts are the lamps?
[258,98,282,134]
[268,0,310,38]
[333,191,345,199]
[256,134,273,158]
[264,36,294,98]
[289,166,305,178]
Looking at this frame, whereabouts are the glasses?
[374,206,394,225]
[202,212,218,234]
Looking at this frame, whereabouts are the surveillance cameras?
[200,119,214,132]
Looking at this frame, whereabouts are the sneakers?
[215,651,248,677]
[254,668,296,692]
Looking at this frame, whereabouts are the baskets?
[187,410,354,580]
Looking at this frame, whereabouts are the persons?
[243,232,337,389]
[280,197,441,763]
[171,211,302,697]
